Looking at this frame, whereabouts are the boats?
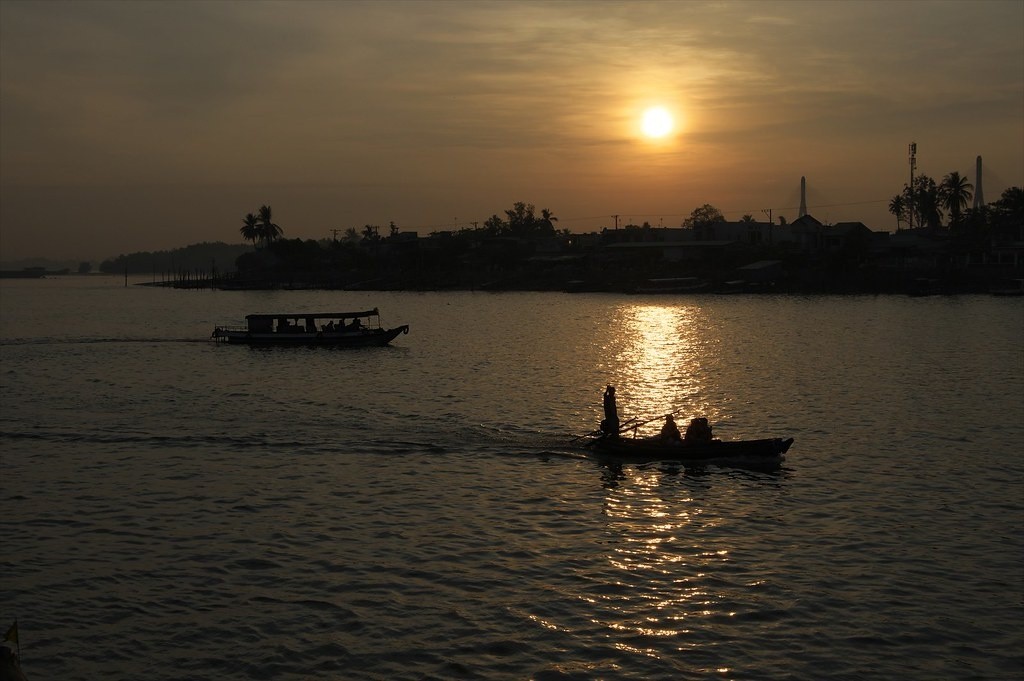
[598,433,793,469]
[211,308,412,344]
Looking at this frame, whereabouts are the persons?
[604,385,620,437]
[685,417,713,439]
[662,414,679,439]
[322,318,364,332]
